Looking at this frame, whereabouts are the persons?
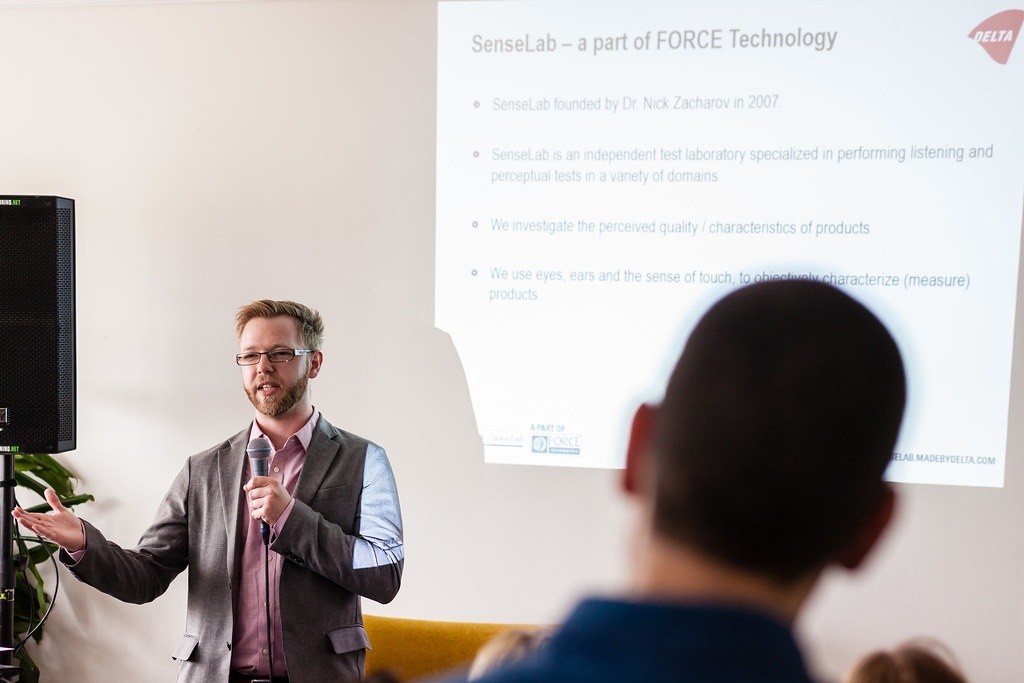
[444,278,971,683]
[12,301,405,683]
[467,624,558,681]
[843,640,965,683]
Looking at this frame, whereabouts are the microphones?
[246,438,272,545]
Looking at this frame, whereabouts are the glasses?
[235,348,313,366]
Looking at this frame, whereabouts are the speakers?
[0,195,78,454]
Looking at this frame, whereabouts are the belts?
[229,674,289,683]
